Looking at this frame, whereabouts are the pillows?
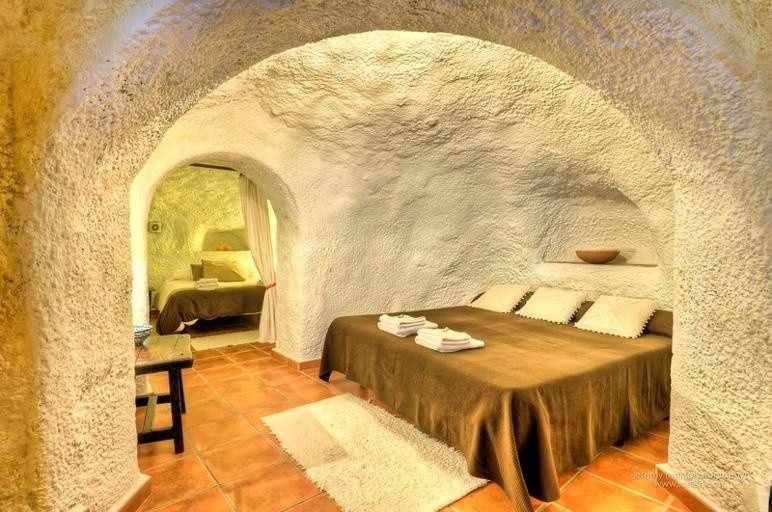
[470,284,658,339]
[172,258,248,282]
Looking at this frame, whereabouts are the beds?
[319,289,673,512]
[156,262,266,335]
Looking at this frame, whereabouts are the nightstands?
[149,288,160,312]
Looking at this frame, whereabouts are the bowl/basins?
[134,326,154,346]
[575,250,620,263]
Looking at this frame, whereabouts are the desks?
[135,331,194,455]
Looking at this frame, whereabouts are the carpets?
[260,393,492,512]
